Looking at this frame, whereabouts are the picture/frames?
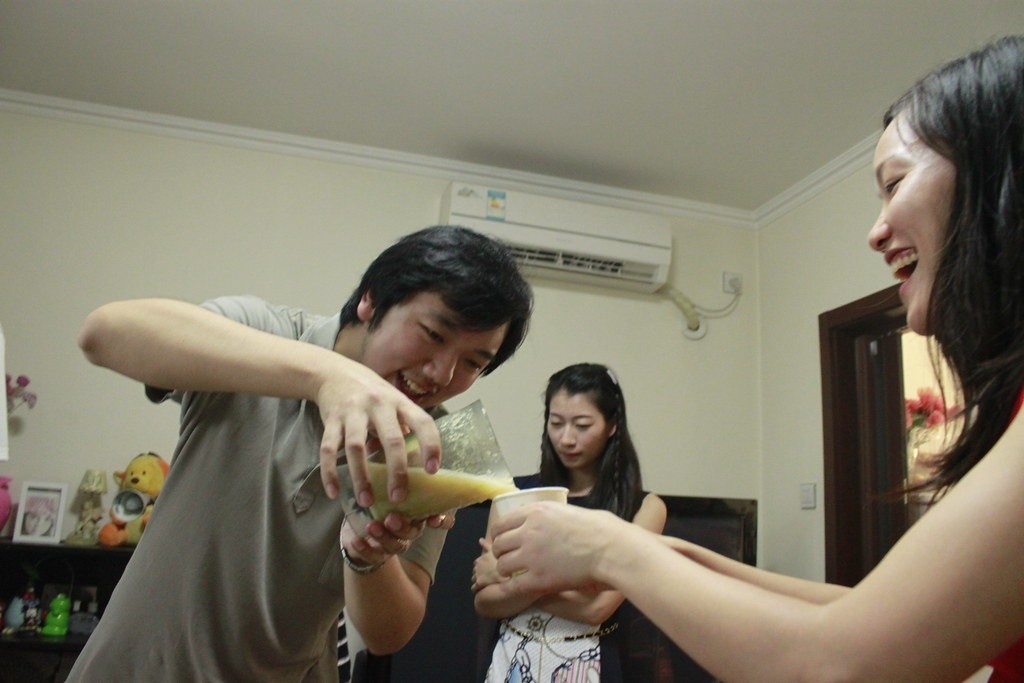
[13,481,67,544]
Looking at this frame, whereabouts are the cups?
[335,400,517,538]
[492,486,570,578]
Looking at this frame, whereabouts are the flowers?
[5,374,39,412]
[904,382,964,449]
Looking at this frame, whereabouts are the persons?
[20,495,57,536]
[485,35,1024,682]
[468,362,670,683]
[61,224,534,683]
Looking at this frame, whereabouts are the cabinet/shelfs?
[0,539,136,683]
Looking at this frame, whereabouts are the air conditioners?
[438,181,671,292]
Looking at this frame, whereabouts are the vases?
[910,446,919,482]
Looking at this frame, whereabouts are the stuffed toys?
[96,451,169,547]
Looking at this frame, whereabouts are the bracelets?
[337,542,388,572]
